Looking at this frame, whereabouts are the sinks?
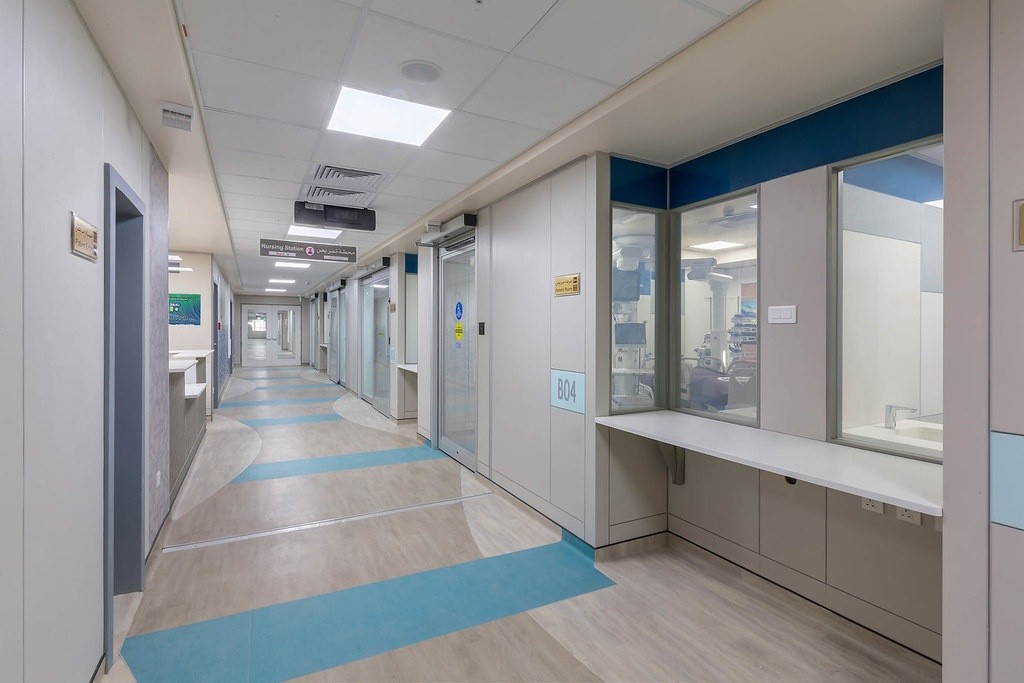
[894,427,943,443]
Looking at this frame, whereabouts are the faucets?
[885,404,917,429]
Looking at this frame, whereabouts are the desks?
[398,363,417,374]
[592,409,944,517]
[611,368,655,374]
[320,343,327,348]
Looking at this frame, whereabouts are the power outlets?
[895,505,921,525]
[860,496,884,515]
[934,517,943,531]
